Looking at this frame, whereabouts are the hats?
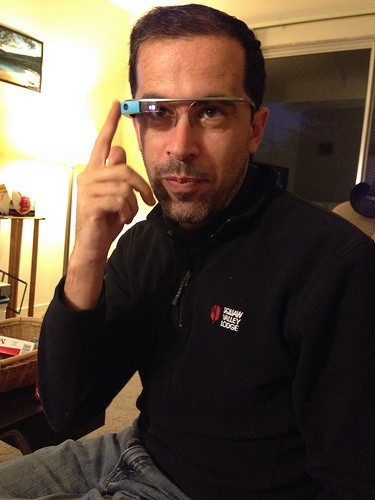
[349,181,375,218]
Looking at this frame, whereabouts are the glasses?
[120,96,257,130]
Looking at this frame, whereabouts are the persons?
[0,4,375,500]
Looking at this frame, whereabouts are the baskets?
[0,317,43,392]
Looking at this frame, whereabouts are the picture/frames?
[0,23,43,93]
